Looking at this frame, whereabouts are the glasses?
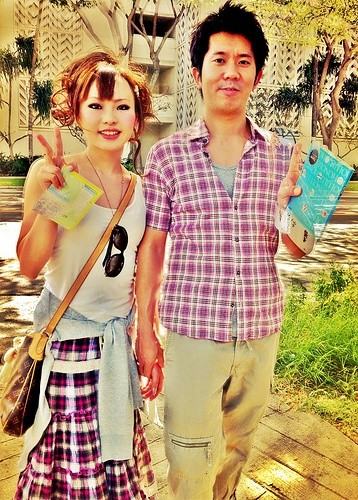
[104,225,129,278]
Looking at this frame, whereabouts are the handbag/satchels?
[0,331,47,436]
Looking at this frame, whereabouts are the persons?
[130,0,314,499]
[8,49,159,500]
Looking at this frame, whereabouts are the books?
[282,142,355,256]
[28,165,104,231]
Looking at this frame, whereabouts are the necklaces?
[85,151,125,226]
